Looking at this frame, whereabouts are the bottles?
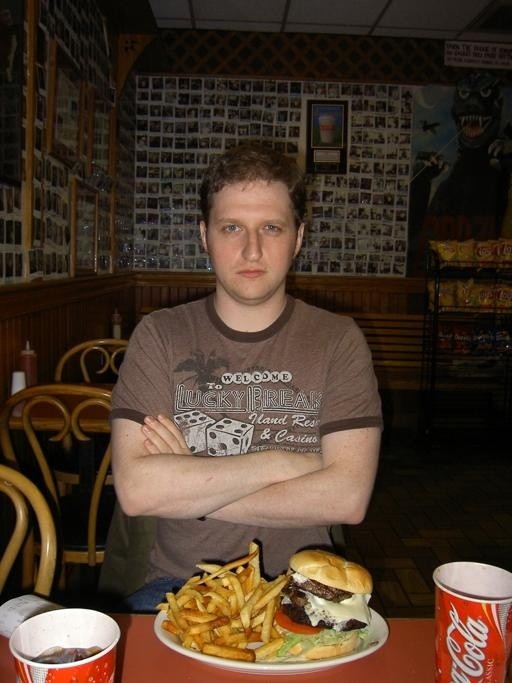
[319,110,336,144]
[20,341,37,388]
[110,308,122,339]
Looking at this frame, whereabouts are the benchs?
[333,313,510,432]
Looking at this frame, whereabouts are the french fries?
[156,542,287,662]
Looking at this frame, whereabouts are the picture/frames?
[44,36,135,275]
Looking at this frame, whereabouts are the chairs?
[1,336,129,596]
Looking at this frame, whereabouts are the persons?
[96,140,384,615]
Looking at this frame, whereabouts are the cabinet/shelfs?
[419,251,511,456]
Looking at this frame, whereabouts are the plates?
[154,595,389,675]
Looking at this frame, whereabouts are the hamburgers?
[273,550,374,660]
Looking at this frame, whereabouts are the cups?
[9,608,120,683]
[432,562,512,683]
